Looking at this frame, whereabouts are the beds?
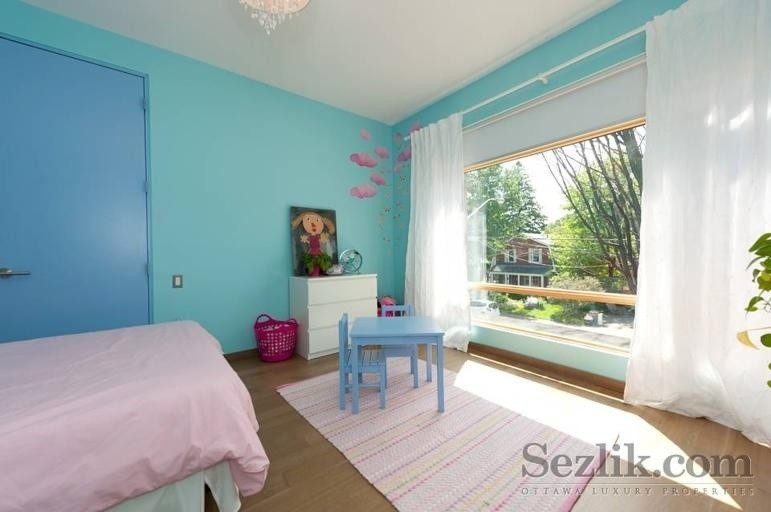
[1,318,270,511]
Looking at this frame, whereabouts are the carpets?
[276,357,622,511]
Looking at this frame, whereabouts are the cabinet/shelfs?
[289,272,379,361]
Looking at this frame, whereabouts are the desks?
[349,314,448,415]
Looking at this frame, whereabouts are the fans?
[338,248,363,274]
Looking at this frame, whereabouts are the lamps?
[235,0,314,33]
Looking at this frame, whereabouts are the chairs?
[380,303,420,388]
[339,312,387,410]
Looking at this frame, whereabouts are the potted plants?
[300,254,333,276]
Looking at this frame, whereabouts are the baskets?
[255,314,299,362]
